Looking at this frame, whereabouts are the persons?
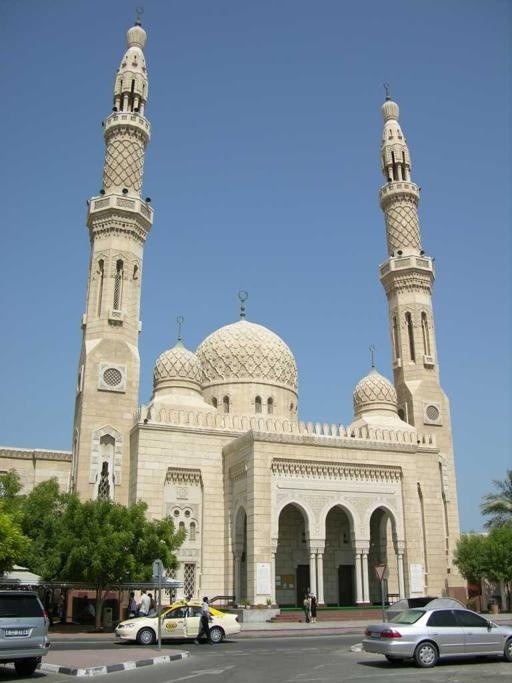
[147,592,156,612]
[310,596,319,622]
[304,586,315,598]
[137,587,150,617]
[56,592,65,617]
[301,594,311,622]
[194,596,214,644]
[128,591,137,615]
[80,595,89,617]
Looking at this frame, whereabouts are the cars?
[115,601,243,644]
[361,606,512,666]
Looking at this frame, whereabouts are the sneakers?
[305,619,317,623]
[194,637,215,647]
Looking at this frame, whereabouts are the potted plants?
[266,598,272,608]
[237,600,251,610]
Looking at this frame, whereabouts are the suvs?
[0,590,53,678]
